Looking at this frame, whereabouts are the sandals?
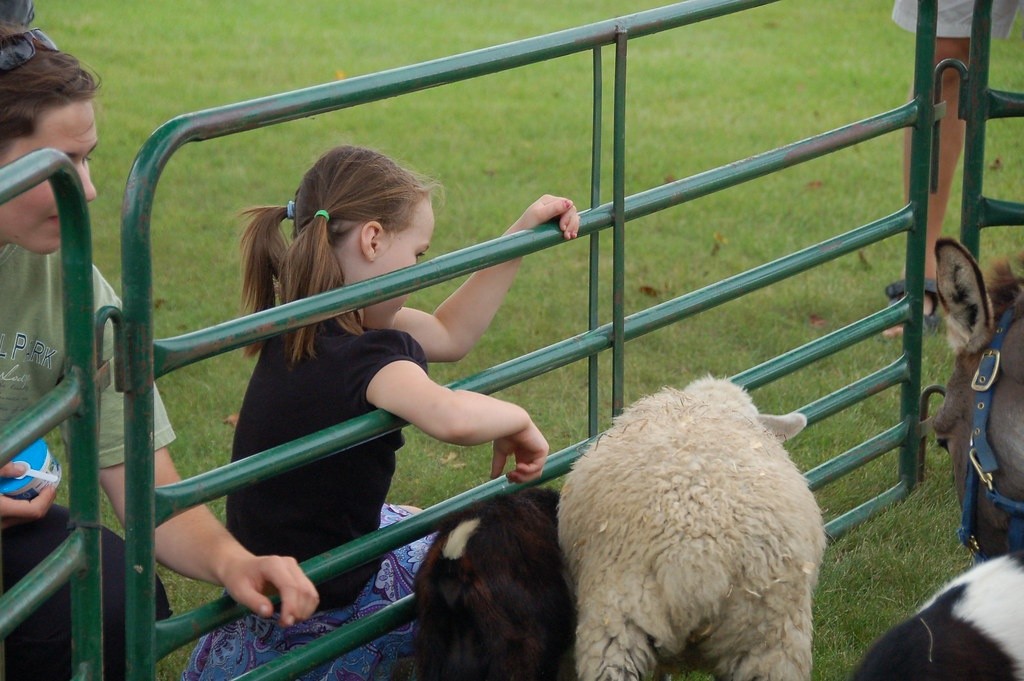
[876,278,943,345]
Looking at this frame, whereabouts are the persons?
[0,0,321,681]
[876,0,1024,345]
[180,144,580,681]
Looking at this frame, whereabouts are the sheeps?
[558,372,829,681]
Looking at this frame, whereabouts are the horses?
[932,235,1024,553]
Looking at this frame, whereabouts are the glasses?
[0,28,59,71]
